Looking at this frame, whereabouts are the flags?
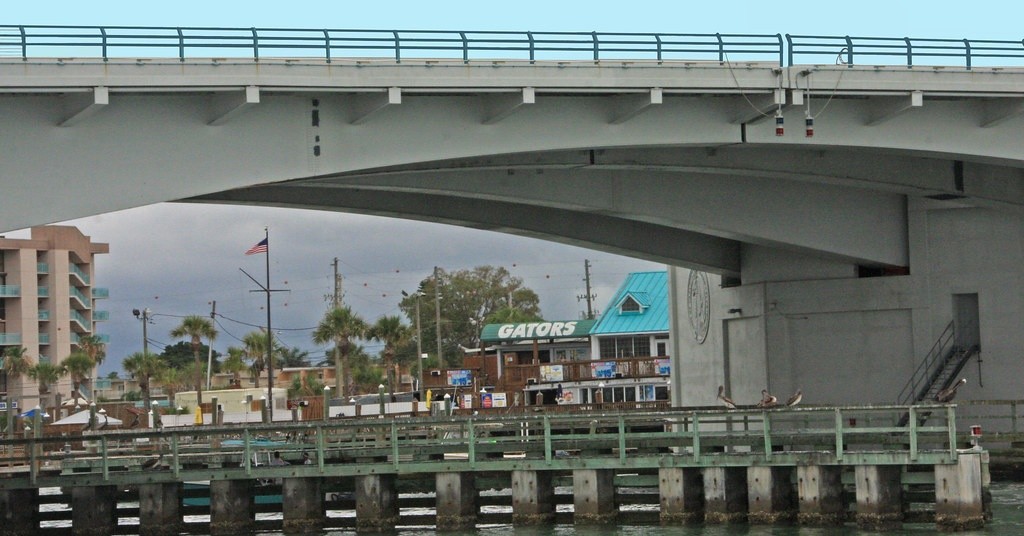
[244,238,267,255]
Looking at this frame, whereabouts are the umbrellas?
[50,409,123,426]
[16,410,49,418]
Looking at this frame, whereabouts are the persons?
[272,451,283,465]
[303,453,311,464]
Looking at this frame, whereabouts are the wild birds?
[933,378,967,401]
[785,388,803,408]
[757,389,779,410]
[717,385,737,410]
